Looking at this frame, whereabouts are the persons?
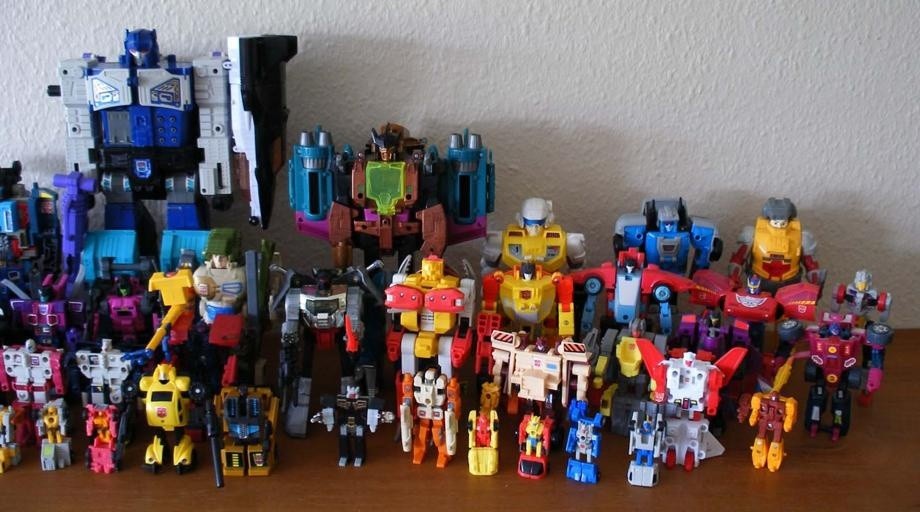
[0,26,893,488]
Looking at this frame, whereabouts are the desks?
[0,327,920,512]
